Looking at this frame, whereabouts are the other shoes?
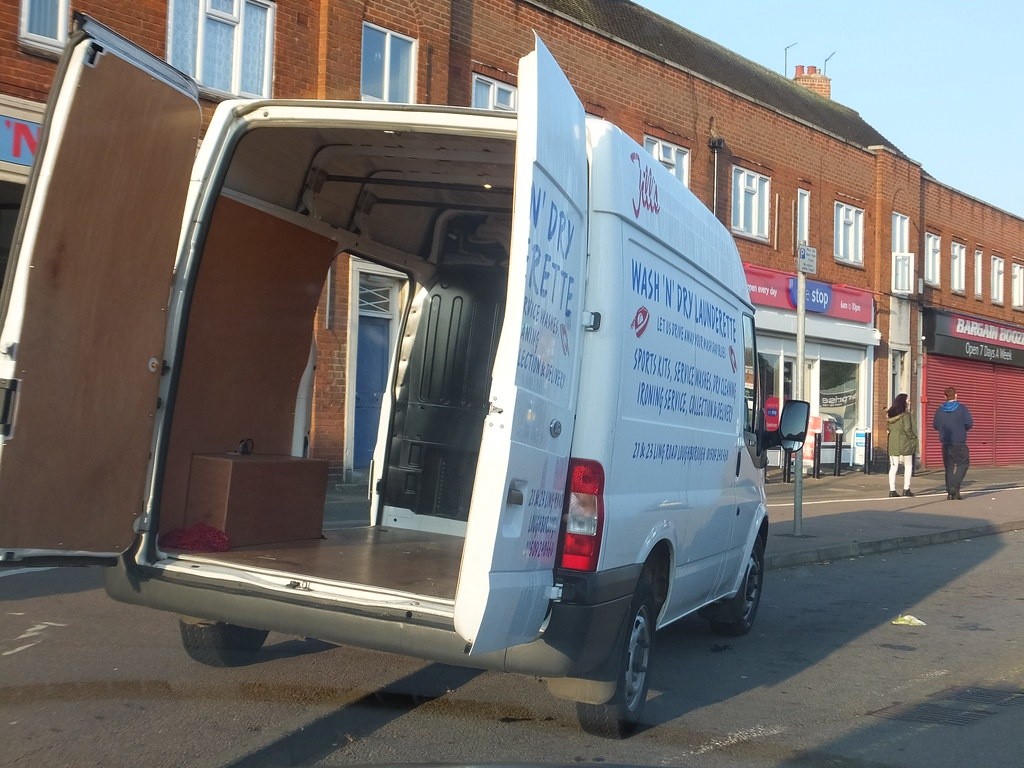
[948,487,961,500]
[903,489,914,496]
[889,491,900,497]
[947,493,954,500]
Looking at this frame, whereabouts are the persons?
[933,387,974,500]
[885,393,916,497]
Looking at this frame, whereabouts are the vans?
[0,13,812,742]
